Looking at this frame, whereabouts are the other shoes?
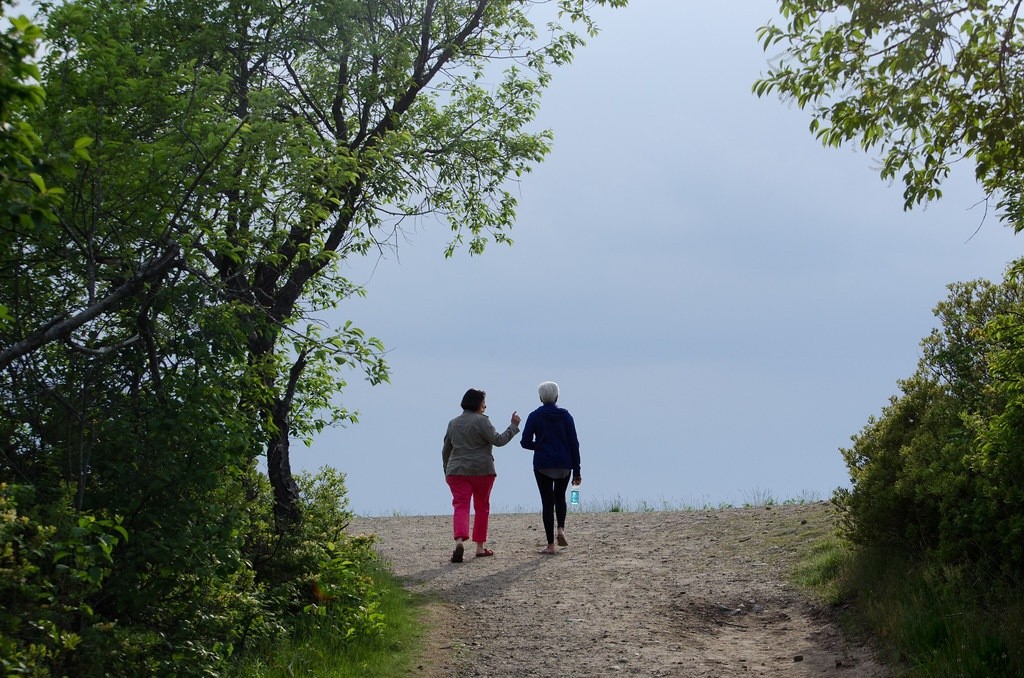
[451,544,464,563]
[557,536,568,546]
[542,548,554,554]
[476,548,493,557]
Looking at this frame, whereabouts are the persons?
[520,381,582,554]
[442,388,521,563]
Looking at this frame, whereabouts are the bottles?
[571,481,580,504]
